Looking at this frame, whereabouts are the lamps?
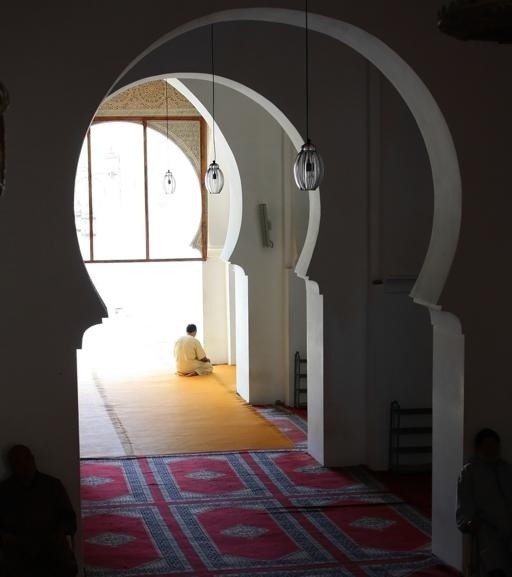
[293,0,324,192]
[162,77,176,196]
[204,25,224,195]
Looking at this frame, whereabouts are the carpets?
[77,363,297,461]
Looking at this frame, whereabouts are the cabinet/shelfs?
[294,351,307,409]
[388,398,432,478]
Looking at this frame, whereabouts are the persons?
[453,427,512,576]
[171,323,214,376]
[0,443,79,577]
